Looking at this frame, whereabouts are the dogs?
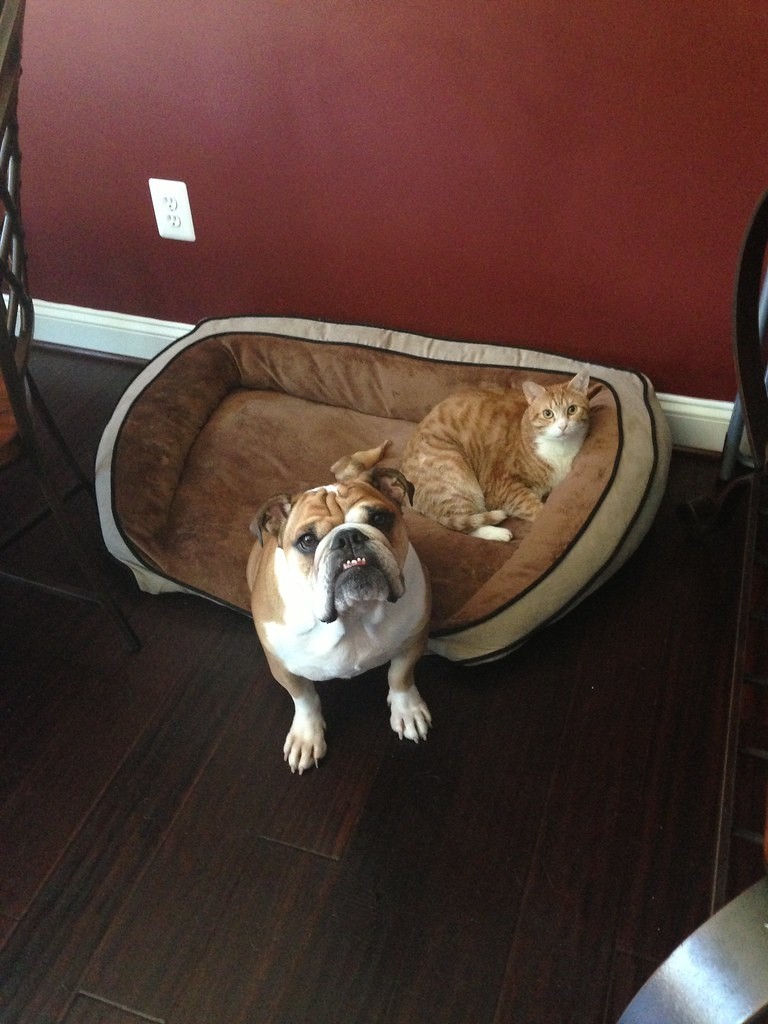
[246,466,433,776]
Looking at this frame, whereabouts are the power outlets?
[148,178,196,243]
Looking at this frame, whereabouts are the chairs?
[0,0,143,658]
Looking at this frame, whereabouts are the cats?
[330,368,590,542]
